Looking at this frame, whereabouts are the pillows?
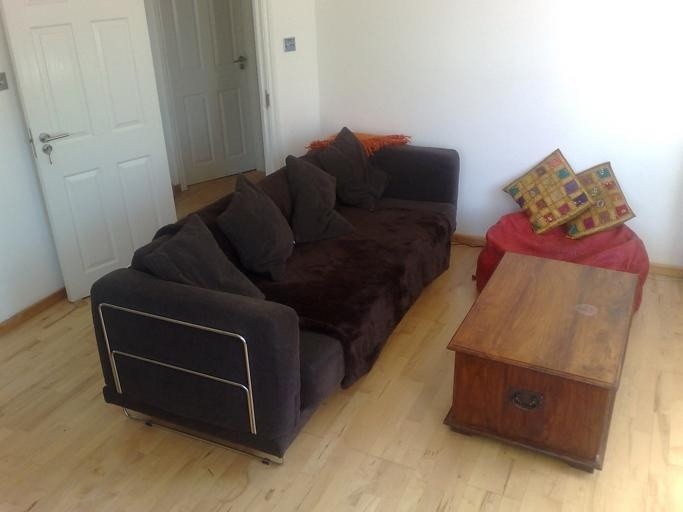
[502,148,596,236]
[317,127,387,214]
[285,153,357,247]
[567,161,635,239]
[143,213,265,305]
[215,173,296,283]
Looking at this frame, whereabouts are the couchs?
[90,133,459,464]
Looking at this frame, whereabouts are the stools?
[475,212,649,315]
